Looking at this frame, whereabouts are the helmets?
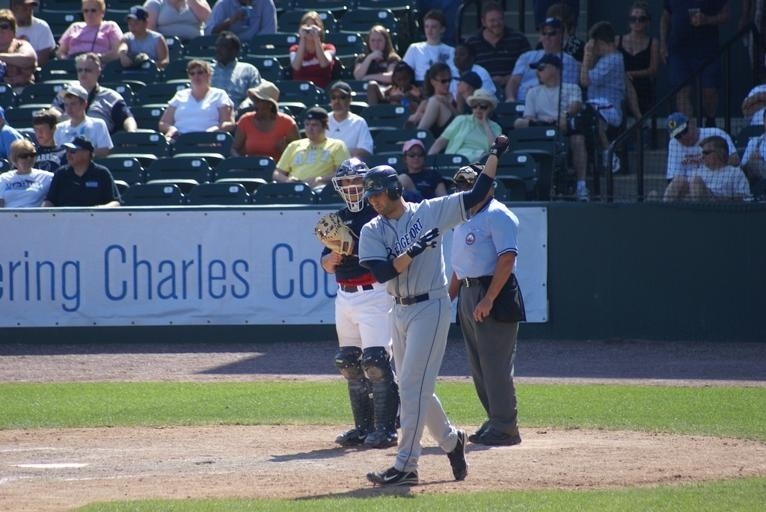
[364,164,403,200]
[330,158,370,212]
[452,163,496,188]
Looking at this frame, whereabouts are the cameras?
[304,28,312,34]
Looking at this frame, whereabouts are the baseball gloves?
[315,213,359,257]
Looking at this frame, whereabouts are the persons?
[320,157,399,448]
[448,164,525,445]
[358,135,509,486]
[1,1,765,202]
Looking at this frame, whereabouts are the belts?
[341,283,373,292]
[462,278,477,287]
[396,292,429,306]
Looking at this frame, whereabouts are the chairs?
[0,0,766,207]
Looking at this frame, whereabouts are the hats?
[248,81,280,113]
[59,85,89,101]
[466,88,498,109]
[452,72,482,88]
[537,15,563,29]
[62,137,94,150]
[126,5,149,21]
[529,53,560,69]
[330,81,351,95]
[665,113,689,137]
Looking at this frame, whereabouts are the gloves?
[490,134,511,157]
[407,227,439,258]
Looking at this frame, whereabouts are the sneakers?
[366,467,419,486]
[575,187,589,202]
[338,431,397,449]
[603,152,620,174]
[449,430,468,481]
[468,422,521,445]
